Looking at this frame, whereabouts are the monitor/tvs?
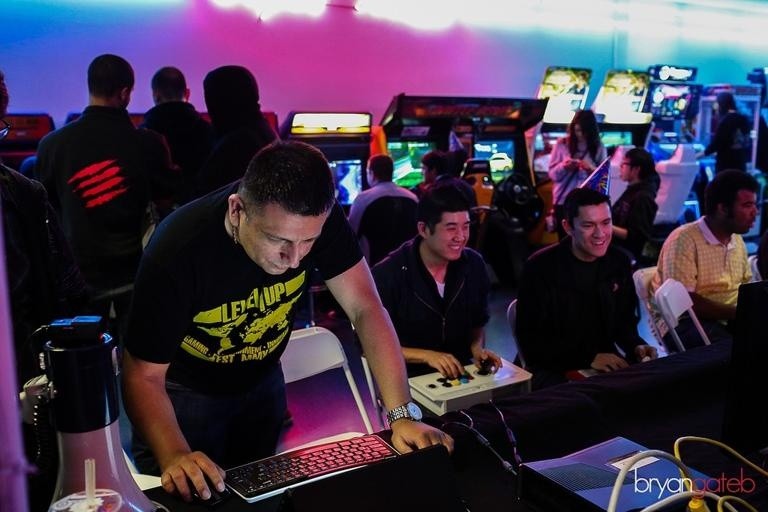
[387,141,438,186]
[599,131,633,155]
[329,159,363,207]
[720,279,768,467]
[536,131,568,151]
[474,139,515,184]
[649,84,692,121]
[280,444,457,512]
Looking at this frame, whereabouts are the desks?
[141,329,768,512]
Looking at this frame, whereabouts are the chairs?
[655,278,712,352]
[360,196,418,270]
[677,191,700,226]
[632,265,668,353]
[359,353,390,432]
[278,327,375,458]
[505,298,536,395]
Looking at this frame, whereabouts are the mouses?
[187,474,230,512]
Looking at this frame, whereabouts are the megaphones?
[18,315,158,512]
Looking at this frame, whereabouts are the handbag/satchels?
[545,216,557,233]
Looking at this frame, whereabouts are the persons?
[512,187,658,397]
[0,54,504,509]
[609,148,660,264]
[647,169,760,355]
[547,109,608,245]
[695,91,753,176]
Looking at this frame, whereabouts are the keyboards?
[222,435,403,498]
